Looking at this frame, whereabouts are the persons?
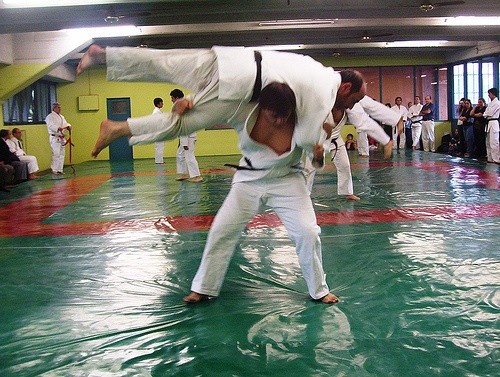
[168,88,204,183]
[353,124,369,158]
[45,101,72,177]
[405,101,415,152]
[346,95,405,161]
[170,81,342,304]
[313,103,359,201]
[483,87,500,165]
[419,95,435,154]
[0,127,41,192]
[407,96,426,151]
[391,96,415,151]
[381,102,395,149]
[471,97,489,162]
[344,133,358,151]
[448,98,474,158]
[75,42,367,158]
[151,98,166,164]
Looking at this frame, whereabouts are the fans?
[390,0,465,12]
[103,5,152,24]
[132,38,170,48]
[338,30,394,40]
[309,49,359,58]
